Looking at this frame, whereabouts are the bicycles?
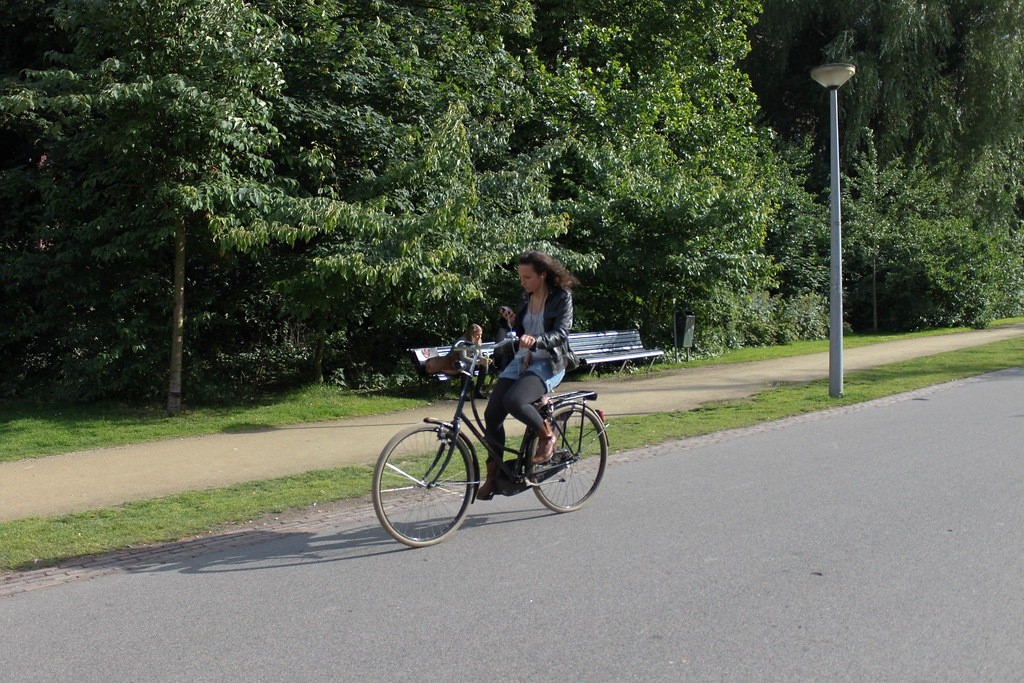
[371,330,611,548]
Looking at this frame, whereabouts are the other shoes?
[473,392,487,399]
[460,395,470,401]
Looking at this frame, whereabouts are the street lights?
[810,62,857,398]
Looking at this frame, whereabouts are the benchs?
[405,327,662,403]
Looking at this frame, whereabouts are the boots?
[530,419,558,464]
[479,457,503,496]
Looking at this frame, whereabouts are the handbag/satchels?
[426,357,449,373]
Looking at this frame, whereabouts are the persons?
[477,250,580,500]
[450,324,490,401]
